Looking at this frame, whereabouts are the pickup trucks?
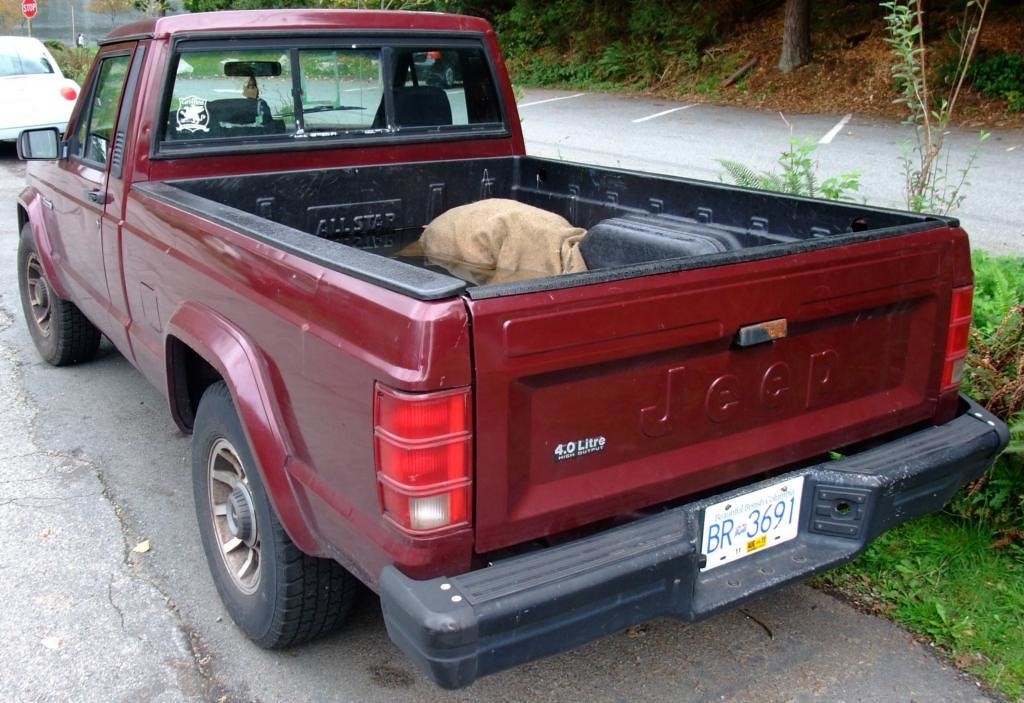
[17,8,1011,690]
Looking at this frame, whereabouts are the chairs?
[164,98,285,141]
[372,86,452,128]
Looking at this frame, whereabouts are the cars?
[0,35,82,142]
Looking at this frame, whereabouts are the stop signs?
[22,0,37,19]
